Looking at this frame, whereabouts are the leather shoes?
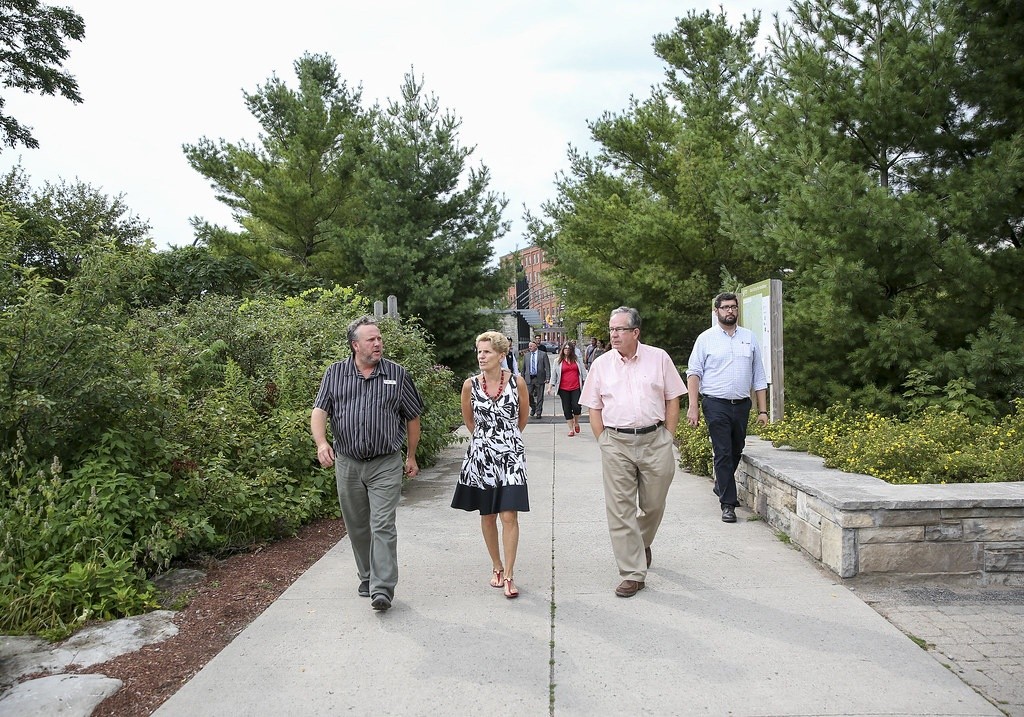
[644,547,652,568]
[615,579,646,597]
[722,505,737,523]
[713,488,741,508]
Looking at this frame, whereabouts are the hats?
[506,336,512,342]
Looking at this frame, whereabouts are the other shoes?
[537,414,541,419]
[530,407,536,416]
[574,424,580,433]
[357,579,370,597]
[568,430,574,436]
[371,593,391,610]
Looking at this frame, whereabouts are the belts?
[529,375,537,378]
[605,421,663,435]
[360,455,379,463]
[703,394,749,405]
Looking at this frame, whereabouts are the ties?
[531,352,536,375]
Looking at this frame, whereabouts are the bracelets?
[758,411,767,415]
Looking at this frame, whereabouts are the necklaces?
[482,370,504,401]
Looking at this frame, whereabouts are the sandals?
[490,565,504,588]
[504,577,518,597]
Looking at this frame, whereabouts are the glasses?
[606,326,637,334]
[563,347,570,350]
[717,305,740,311]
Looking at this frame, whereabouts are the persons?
[503,336,612,438]
[311,317,425,611]
[578,306,689,597]
[687,293,768,524]
[450,329,534,598]
